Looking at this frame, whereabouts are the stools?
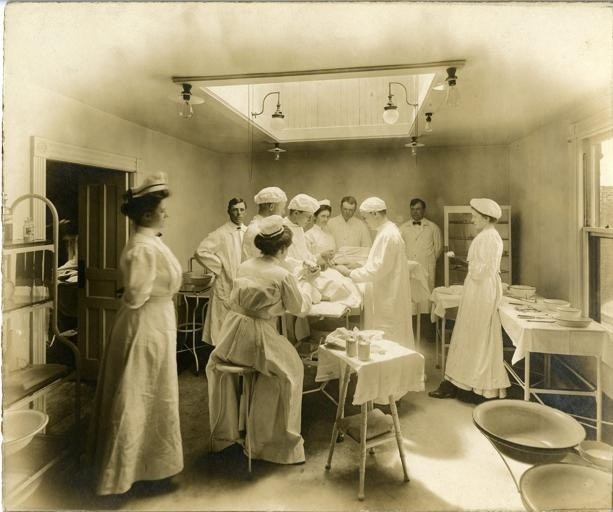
[206,362,259,474]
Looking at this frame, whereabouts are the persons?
[429,198,509,401]
[85,170,185,500]
[196,187,441,465]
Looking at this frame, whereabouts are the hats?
[470,197,501,221]
[359,197,386,215]
[253,186,288,205]
[256,215,284,237]
[131,171,167,199]
[318,199,330,207]
[287,193,320,213]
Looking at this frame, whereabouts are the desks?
[175,287,214,377]
[316,337,424,500]
[498,293,604,444]
[430,285,465,385]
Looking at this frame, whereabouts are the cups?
[346,336,357,357]
[358,341,370,361]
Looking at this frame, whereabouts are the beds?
[278,261,427,407]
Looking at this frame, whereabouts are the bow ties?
[412,221,421,226]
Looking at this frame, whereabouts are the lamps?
[177,84,195,119]
[267,144,287,161]
[253,90,287,133]
[383,81,417,124]
[445,66,460,108]
[405,138,424,156]
[424,113,433,133]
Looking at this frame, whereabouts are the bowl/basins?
[473,399,587,464]
[190,277,210,286]
[559,309,581,319]
[560,320,593,327]
[543,299,569,311]
[4,409,48,458]
[519,463,613,511]
[580,439,613,468]
[510,285,536,297]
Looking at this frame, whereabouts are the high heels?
[428,381,457,399]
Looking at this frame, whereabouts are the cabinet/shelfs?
[1,193,82,511]
[444,204,512,289]
